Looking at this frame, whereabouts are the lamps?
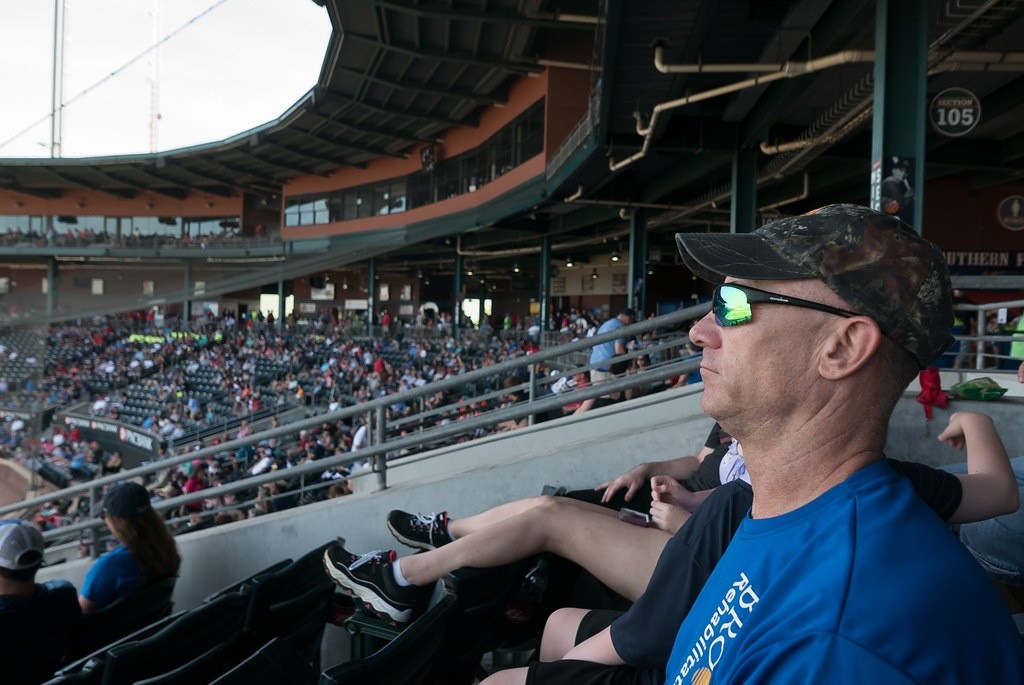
[611,250,620,261]
[567,256,573,267]
[417,271,422,278]
[593,268,597,278]
[514,262,519,272]
[648,266,653,275]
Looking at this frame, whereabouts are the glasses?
[711,283,865,328]
[98,510,106,520]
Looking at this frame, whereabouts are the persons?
[1,303,703,559]
[0,482,180,685]
[3,228,250,248]
[418,166,516,203]
[880,158,916,217]
[323,204,1024,684]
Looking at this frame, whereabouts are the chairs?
[0,306,1024,685]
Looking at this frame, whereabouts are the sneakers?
[322,544,421,627]
[386,509,459,578]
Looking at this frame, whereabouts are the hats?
[621,308,636,321]
[892,159,911,170]
[0,523,44,570]
[675,202,954,363]
